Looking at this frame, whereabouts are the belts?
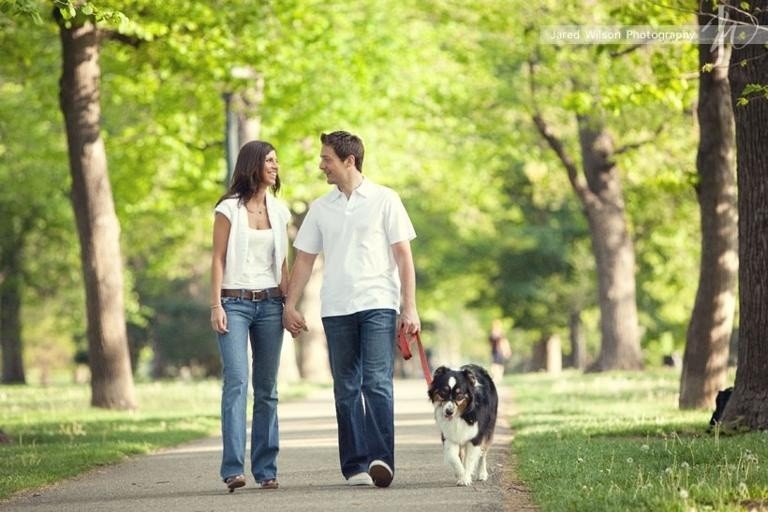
[220,287,283,302]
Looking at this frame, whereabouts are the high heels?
[259,478,278,488]
[227,474,245,492]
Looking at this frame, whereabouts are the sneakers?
[369,460,394,487]
[349,471,373,486]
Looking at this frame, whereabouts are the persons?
[209,140,309,492]
[282,131,422,488]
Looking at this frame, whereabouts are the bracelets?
[210,304,223,309]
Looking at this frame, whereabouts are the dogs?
[427,363,499,486]
